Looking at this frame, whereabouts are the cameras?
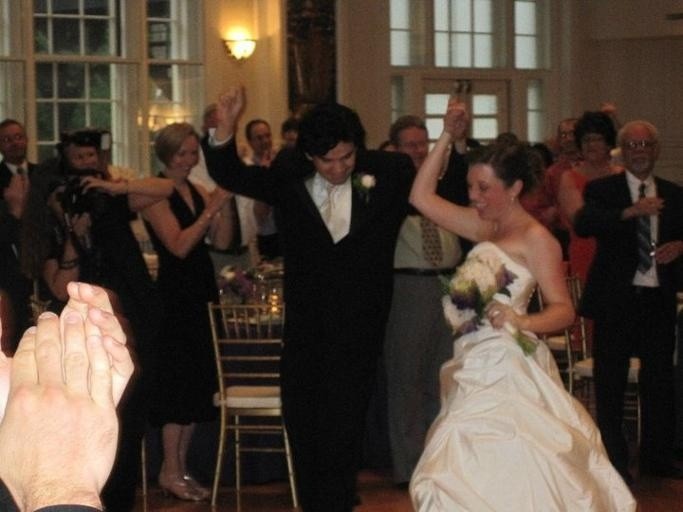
[56,174,85,214]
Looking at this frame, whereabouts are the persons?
[387,115,467,491]
[1,118,42,236]
[241,119,283,258]
[187,104,258,278]
[0,281,135,509]
[138,122,231,461]
[44,127,144,222]
[543,113,574,206]
[561,113,623,365]
[32,177,177,316]
[572,119,681,487]
[201,85,462,512]
[279,113,300,148]
[409,106,637,512]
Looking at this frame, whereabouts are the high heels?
[177,465,211,498]
[158,464,206,501]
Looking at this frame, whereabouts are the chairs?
[563,275,643,442]
[140,430,147,494]
[535,280,578,374]
[205,301,299,509]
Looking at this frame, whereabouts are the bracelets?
[202,208,213,220]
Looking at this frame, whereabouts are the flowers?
[215,263,262,298]
[436,262,543,357]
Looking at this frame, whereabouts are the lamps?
[222,31,258,65]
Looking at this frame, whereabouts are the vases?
[219,294,243,316]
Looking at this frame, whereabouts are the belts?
[629,284,663,293]
[392,267,457,277]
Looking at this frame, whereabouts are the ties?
[18,166,25,177]
[320,185,338,225]
[637,183,653,275]
[420,214,444,267]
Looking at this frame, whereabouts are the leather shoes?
[638,465,682,479]
[623,471,634,485]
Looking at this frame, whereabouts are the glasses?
[559,131,574,138]
[581,136,606,143]
[622,139,656,151]
